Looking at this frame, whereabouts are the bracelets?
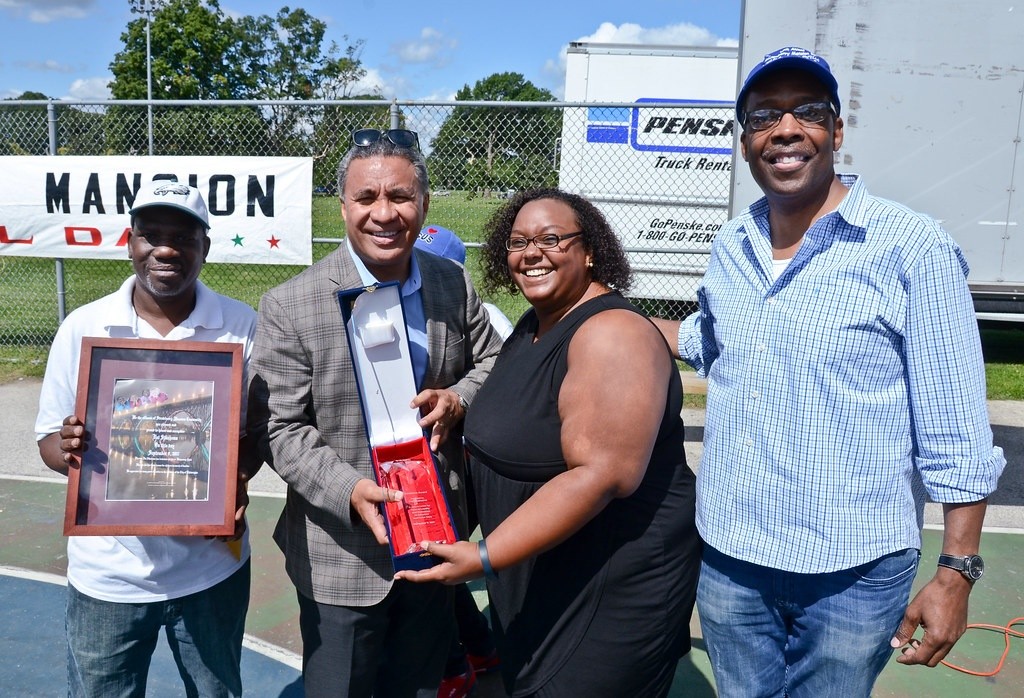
[479,539,492,574]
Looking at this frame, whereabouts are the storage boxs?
[337,280,460,573]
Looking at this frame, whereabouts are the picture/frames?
[62,336,243,537]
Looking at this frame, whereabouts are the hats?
[736,47,841,128]
[129,179,210,233]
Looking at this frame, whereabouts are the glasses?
[741,102,836,130]
[348,128,421,155]
[506,230,587,252]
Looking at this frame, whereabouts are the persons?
[245,134,505,698]
[646,44,1008,698]
[34,180,258,698]
[393,187,702,698]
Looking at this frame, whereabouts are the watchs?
[459,397,469,407]
[937,554,985,584]
